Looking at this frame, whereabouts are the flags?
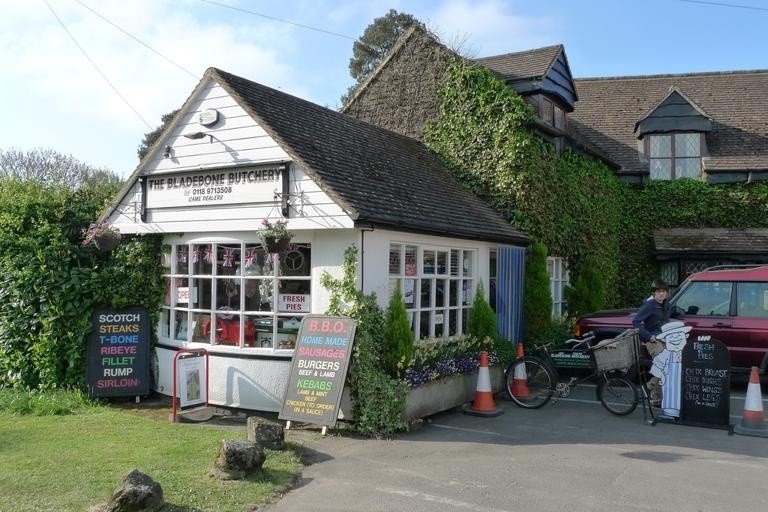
[177,244,187,263]
[192,245,199,263]
[201,244,211,262]
[221,246,235,268]
[265,247,272,264]
[244,248,256,270]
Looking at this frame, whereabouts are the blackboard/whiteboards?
[680,338,731,430]
[278,314,356,427]
[85,306,151,399]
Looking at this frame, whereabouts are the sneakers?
[643,384,653,399]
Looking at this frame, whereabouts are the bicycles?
[504,331,639,415]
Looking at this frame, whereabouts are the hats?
[649,279,668,290]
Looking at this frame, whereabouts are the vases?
[94,239,119,252]
[260,239,290,252]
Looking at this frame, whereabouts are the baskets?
[589,333,642,373]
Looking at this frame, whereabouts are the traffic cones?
[460,350,503,418]
[497,341,537,402]
[733,366,768,438]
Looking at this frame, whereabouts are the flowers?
[384,333,515,392]
[256,218,296,243]
[80,223,123,247]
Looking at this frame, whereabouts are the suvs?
[572,264,768,387]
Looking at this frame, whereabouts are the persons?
[631,278,673,407]
[646,320,710,422]
